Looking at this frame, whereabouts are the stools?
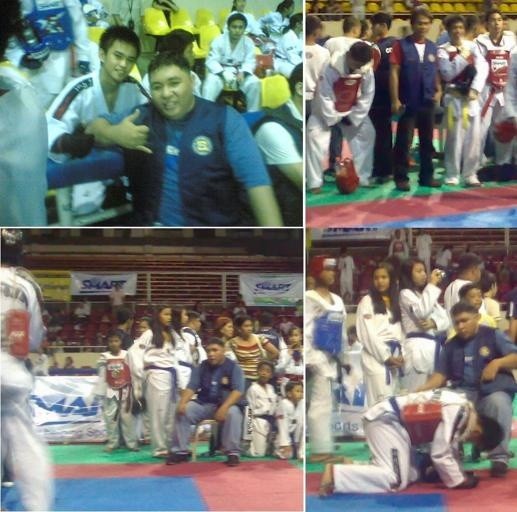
[187,419,221,461]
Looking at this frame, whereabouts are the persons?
[411,300,516,478]
[0,229,54,511]
[24,280,304,466]
[316,385,506,498]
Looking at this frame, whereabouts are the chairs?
[306,0,517,20]
[39,306,303,376]
[307,247,517,308]
[0,5,303,113]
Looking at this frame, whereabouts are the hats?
[214,317,229,329]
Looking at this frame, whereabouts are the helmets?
[335,157,360,193]
[309,254,337,281]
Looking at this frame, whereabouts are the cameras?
[433,269,446,279]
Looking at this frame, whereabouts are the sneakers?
[419,179,440,188]
[168,454,186,464]
[228,454,240,466]
[394,179,410,191]
[490,460,507,478]
